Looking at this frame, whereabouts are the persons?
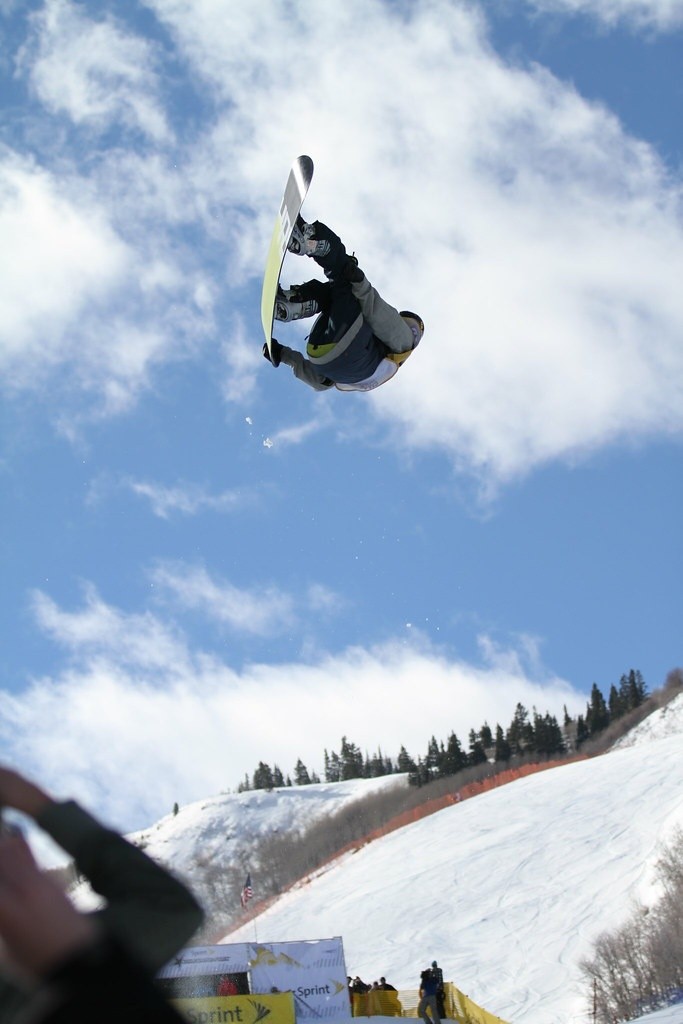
[261,211,424,391]
[0,764,204,1024]
[347,960,447,1024]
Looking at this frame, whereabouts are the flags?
[240,872,253,912]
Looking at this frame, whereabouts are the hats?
[432,960,437,967]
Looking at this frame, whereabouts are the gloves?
[323,259,365,283]
[262,339,283,368]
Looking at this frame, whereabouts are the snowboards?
[260,155,314,368]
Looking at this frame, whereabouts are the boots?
[275,288,318,323]
[288,215,330,257]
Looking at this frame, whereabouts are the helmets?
[400,311,424,335]
